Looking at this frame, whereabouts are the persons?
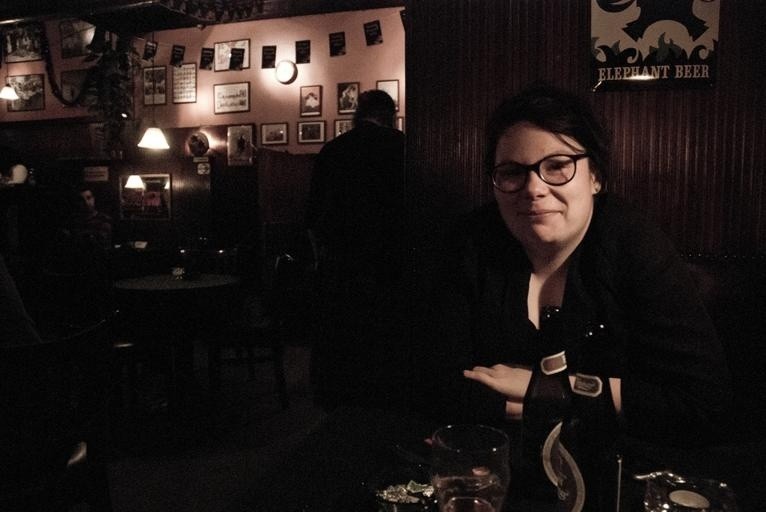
[65,183,113,247]
[302,87,428,413]
[409,80,726,440]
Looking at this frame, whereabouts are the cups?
[432,424,511,510]
[662,478,737,512]
[645,469,685,511]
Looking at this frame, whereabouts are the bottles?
[557,317,615,511]
[518,304,572,512]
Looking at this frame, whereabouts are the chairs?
[2,329,115,508]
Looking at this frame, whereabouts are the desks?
[110,270,242,412]
[218,402,764,510]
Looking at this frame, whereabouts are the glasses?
[491,152,590,193]
